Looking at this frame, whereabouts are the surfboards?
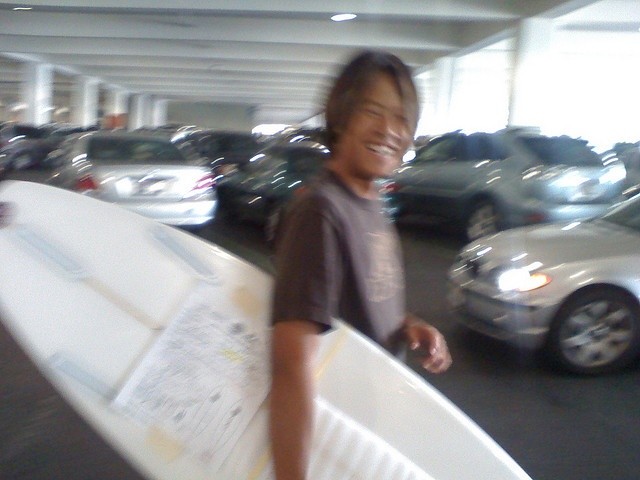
[0,180,532,480]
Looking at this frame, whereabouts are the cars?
[42,127,219,230]
[0,121,40,176]
[441,194,640,375]
[215,129,409,247]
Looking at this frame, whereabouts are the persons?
[267,49,453,479]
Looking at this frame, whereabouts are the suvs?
[397,130,629,243]
